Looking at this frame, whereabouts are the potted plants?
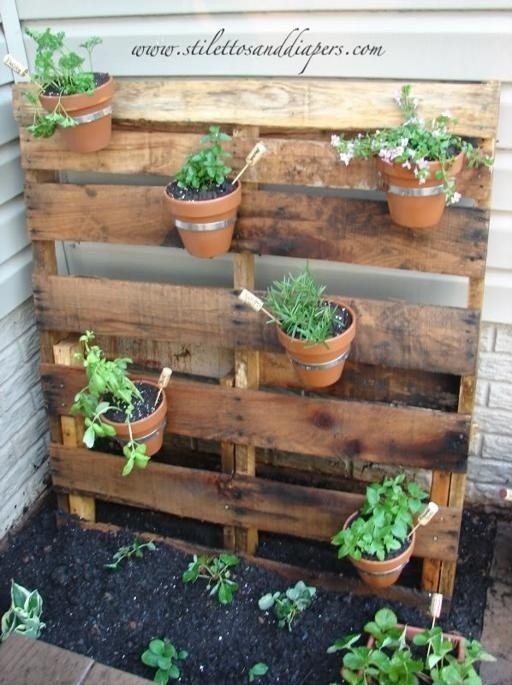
[237,258,357,388]
[2,26,118,154]
[325,592,498,685]
[330,473,439,590]
[163,125,267,258]
[68,329,173,476]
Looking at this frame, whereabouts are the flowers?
[329,85,496,207]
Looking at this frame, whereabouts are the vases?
[374,145,464,228]
